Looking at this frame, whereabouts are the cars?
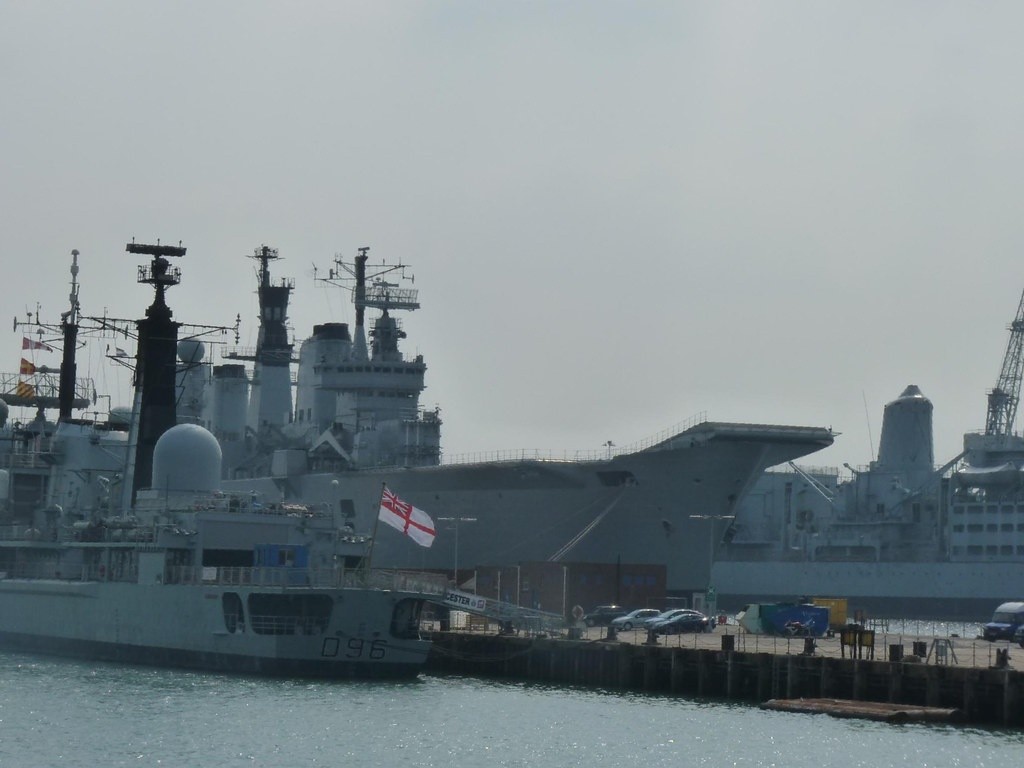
[611,608,661,631]
[582,605,629,627]
[656,614,716,635]
[644,608,703,632]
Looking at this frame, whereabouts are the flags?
[22,337,53,352]
[17,382,34,397]
[20,358,36,375]
[378,486,435,547]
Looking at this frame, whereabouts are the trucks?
[983,602,1024,649]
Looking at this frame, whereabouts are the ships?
[707,383,1024,620]
[0,234,844,681]
